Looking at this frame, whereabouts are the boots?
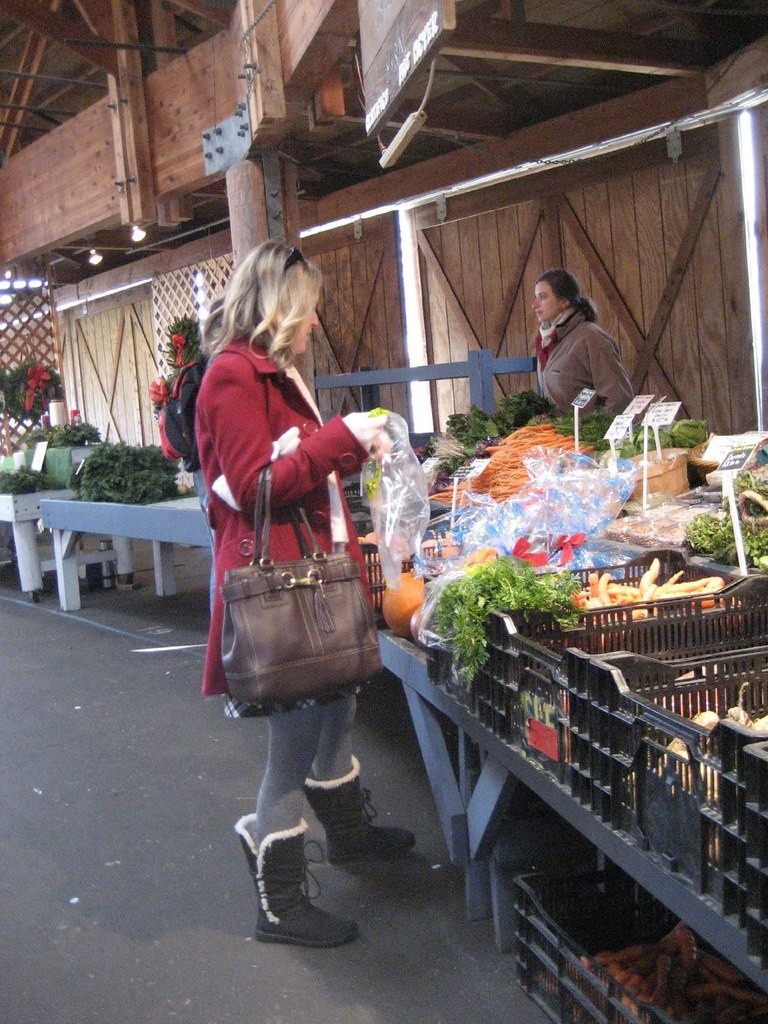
[234,812,359,946]
[303,755,414,864]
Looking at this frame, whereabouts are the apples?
[177,484,189,495]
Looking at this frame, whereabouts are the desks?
[374,629,768,1024]
[44,497,221,611]
[0,489,98,606]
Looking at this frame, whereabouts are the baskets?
[738,490,768,532]
[688,441,719,483]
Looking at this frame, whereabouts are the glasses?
[283,246,304,270]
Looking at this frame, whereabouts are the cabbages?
[597,417,709,464]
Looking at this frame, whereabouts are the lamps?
[379,57,437,168]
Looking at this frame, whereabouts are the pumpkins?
[381,567,427,646]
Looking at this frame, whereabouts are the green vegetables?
[436,556,591,681]
[683,466,768,573]
[423,389,560,470]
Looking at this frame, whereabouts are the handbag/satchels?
[220,466,383,702]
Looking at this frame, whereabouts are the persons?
[531,268,636,418]
[159,240,416,950]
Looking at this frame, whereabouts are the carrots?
[533,920,768,1024]
[428,424,598,507]
[530,556,742,651]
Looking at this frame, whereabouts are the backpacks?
[160,360,198,461]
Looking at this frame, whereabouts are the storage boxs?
[342,450,768,1024]
